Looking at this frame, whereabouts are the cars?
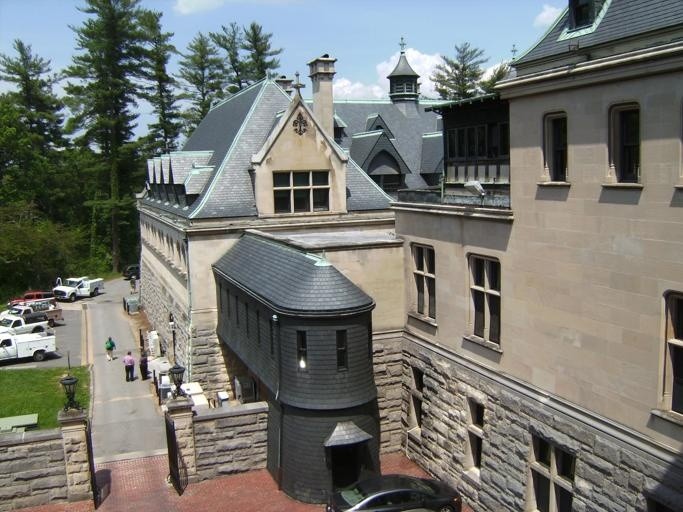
[325,473,463,512]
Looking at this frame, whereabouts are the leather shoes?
[108,358,146,382]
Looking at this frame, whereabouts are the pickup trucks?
[0,300,64,362]
[52,276,105,303]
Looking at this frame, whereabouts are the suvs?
[7,289,56,307]
[122,264,140,280]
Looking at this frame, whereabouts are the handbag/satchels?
[105,343,111,349]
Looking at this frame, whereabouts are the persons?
[123,351,134,382]
[48,303,55,327]
[130,276,136,295]
[105,336,115,361]
[140,352,148,380]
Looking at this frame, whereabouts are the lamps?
[168,361,187,399]
[60,373,79,411]
[464,180,486,200]
